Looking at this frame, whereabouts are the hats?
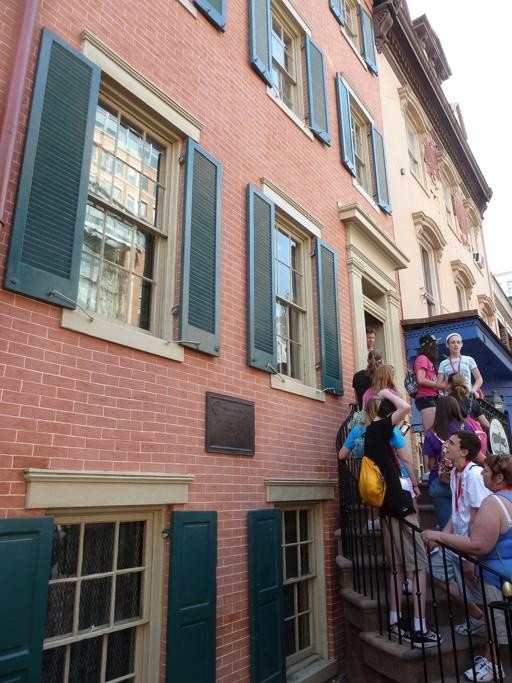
[419,334,441,347]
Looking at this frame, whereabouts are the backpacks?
[358,456,387,507]
[404,358,419,399]
[463,399,487,464]
[430,428,453,484]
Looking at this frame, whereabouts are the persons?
[420,453,511,681]
[338,331,490,649]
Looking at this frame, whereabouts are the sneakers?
[403,579,412,594]
[464,656,505,682]
[368,516,380,531]
[391,618,443,648]
[455,616,485,635]
[422,472,430,482]
